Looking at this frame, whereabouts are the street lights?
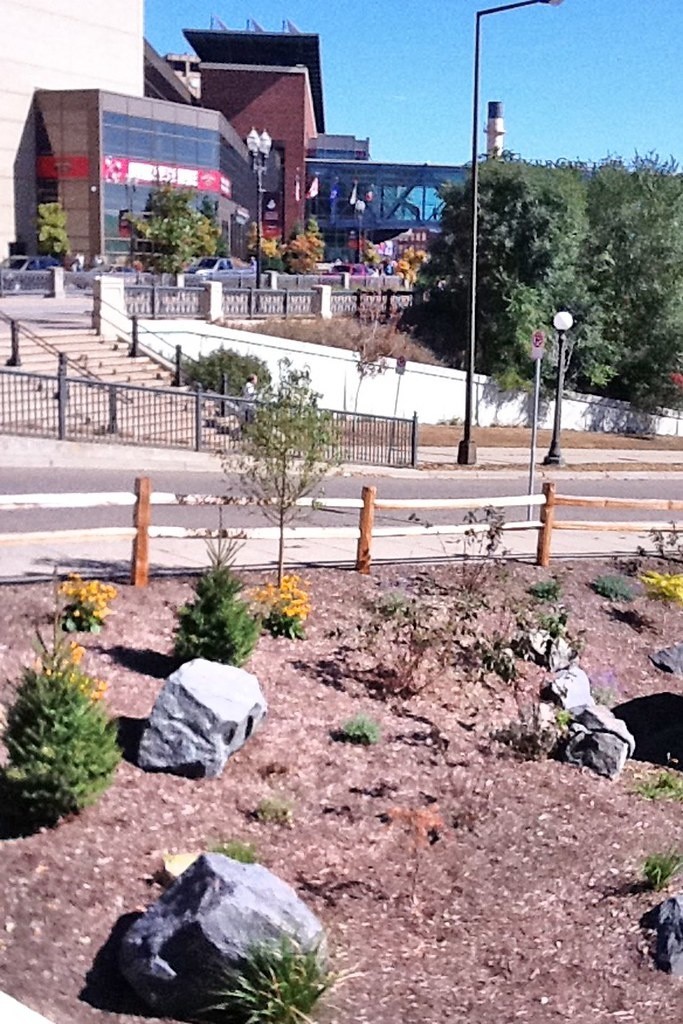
[355,200,367,264]
[245,125,272,315]
[456,1,567,466]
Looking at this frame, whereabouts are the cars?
[319,262,378,290]
[73,263,149,288]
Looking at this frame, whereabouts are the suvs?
[0,254,71,297]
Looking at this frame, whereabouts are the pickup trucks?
[182,255,255,289]
